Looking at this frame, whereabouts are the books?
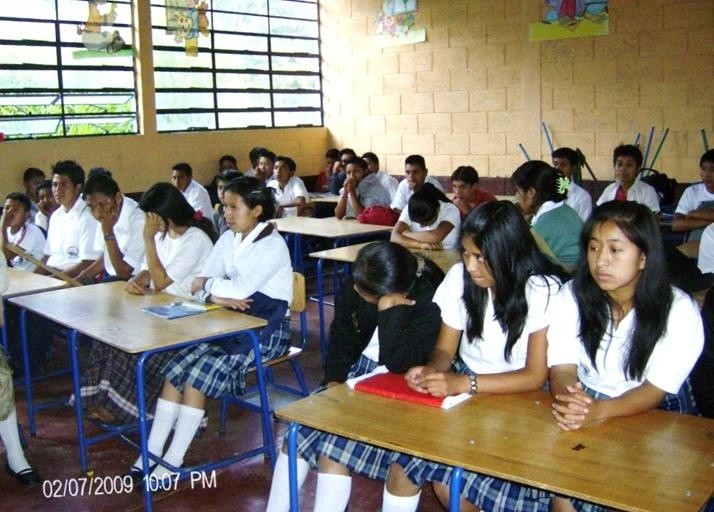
[142,298,225,319]
[348,363,472,410]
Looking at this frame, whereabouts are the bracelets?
[104,234,115,241]
[468,373,478,395]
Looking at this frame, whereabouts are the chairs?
[241,273,308,413]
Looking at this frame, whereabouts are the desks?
[271,367,713,508]
[270,216,393,306]
[1,262,68,386]
[311,241,461,361]
[6,281,277,508]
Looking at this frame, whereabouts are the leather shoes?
[6,460,41,489]
[140,463,186,495]
[118,453,164,483]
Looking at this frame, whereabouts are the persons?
[313,149,340,194]
[448,166,498,218]
[213,168,245,237]
[254,151,276,186]
[256,148,268,163]
[381,199,549,512]
[0,249,42,486]
[328,148,356,194]
[120,176,293,486]
[264,241,446,512]
[210,154,239,201]
[168,163,218,236]
[697,219,714,284]
[265,156,309,218]
[510,159,587,275]
[334,158,384,222]
[72,174,148,285]
[594,143,662,215]
[86,167,113,181]
[244,147,261,176]
[670,150,714,232]
[545,198,706,512]
[0,192,45,348]
[361,152,398,205]
[390,154,445,215]
[34,179,59,231]
[64,181,214,427]
[549,147,592,223]
[389,182,461,252]
[22,167,46,201]
[10,159,98,381]
